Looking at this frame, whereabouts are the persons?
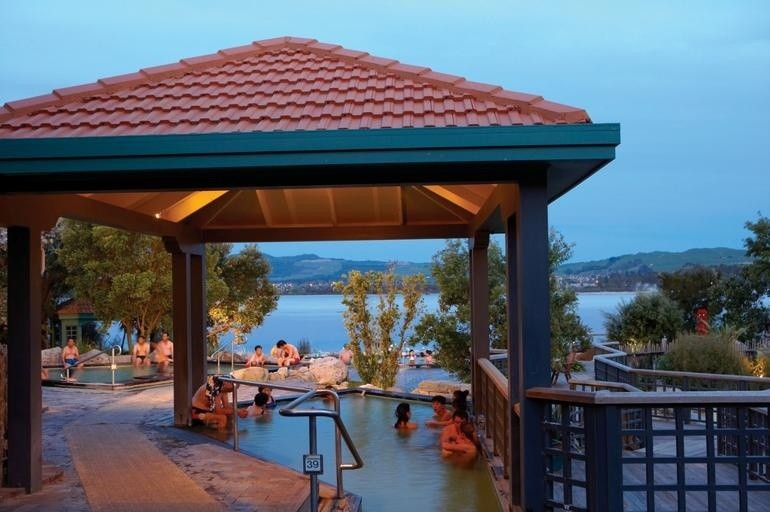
[154,332,175,362]
[339,343,354,365]
[258,387,277,409]
[424,389,480,468]
[61,338,84,372]
[249,345,271,368]
[408,349,416,368]
[41,363,49,380]
[246,392,270,418]
[394,402,418,429]
[192,375,248,440]
[276,339,300,369]
[156,359,170,374]
[424,349,433,368]
[695,300,711,335]
[300,354,306,361]
[64,368,77,381]
[323,384,338,400]
[131,336,151,367]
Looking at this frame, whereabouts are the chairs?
[551,351,576,386]
